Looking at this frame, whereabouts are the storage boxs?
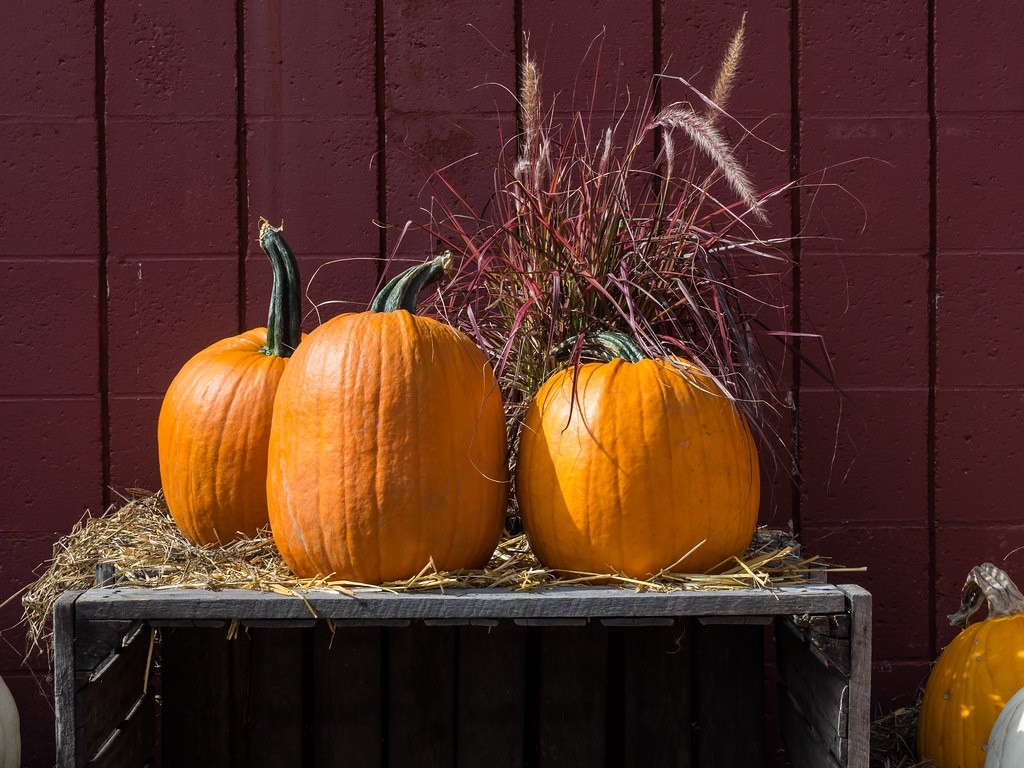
[54,573,871,768]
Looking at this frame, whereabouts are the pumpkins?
[515,330,760,585]
[157,218,508,586]
[917,563,1024,768]
